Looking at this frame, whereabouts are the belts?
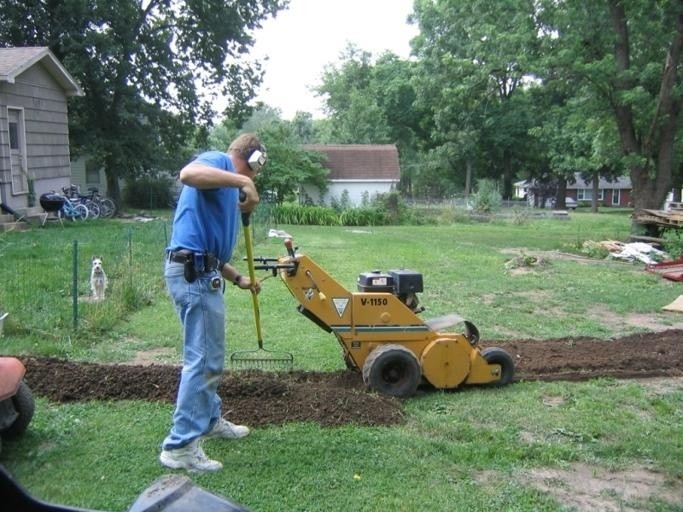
[166,252,224,271]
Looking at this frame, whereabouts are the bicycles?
[50,184,117,223]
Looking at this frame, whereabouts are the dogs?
[88,255,108,300]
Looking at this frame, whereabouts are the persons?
[158,131,267,474]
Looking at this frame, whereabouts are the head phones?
[246,143,268,171]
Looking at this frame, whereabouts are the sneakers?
[199,415,250,440]
[159,437,223,471]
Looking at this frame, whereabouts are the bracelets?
[232,275,242,288]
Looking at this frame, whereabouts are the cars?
[550,196,578,211]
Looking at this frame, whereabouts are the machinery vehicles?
[244,239,514,397]
